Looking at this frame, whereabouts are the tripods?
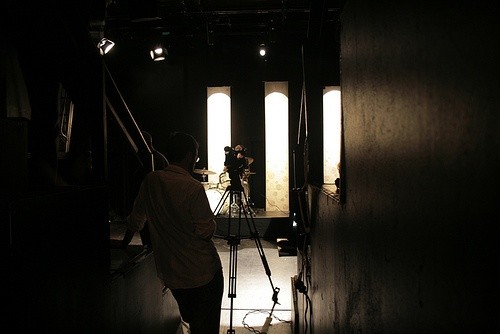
[213,175,280,334]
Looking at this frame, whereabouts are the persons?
[132,130,170,251]
[129,134,224,334]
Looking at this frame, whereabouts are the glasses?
[191,155,200,163]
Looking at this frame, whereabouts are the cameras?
[224,147,247,175]
